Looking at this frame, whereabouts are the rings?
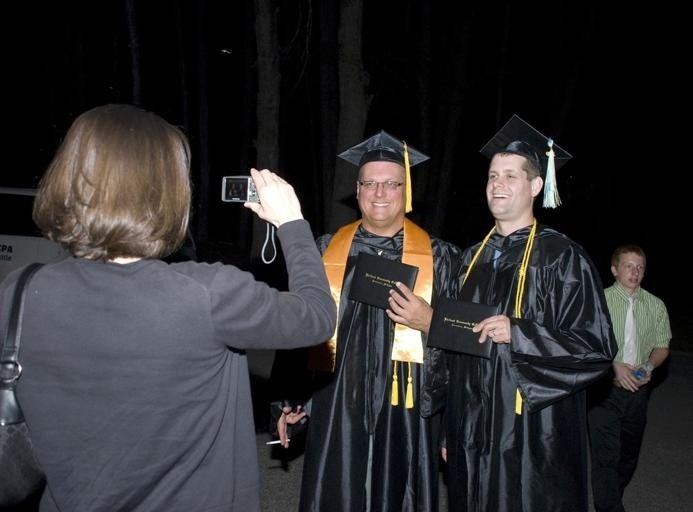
[492,329,496,336]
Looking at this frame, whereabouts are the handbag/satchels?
[0,384,44,507]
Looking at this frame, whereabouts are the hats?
[336,130,430,214]
[480,114,573,209]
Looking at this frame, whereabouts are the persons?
[0,100,339,511]
[436,111,619,511]
[585,233,673,511]
[268,126,470,510]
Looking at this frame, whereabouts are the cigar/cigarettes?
[265,438,290,448]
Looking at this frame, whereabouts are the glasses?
[358,180,404,191]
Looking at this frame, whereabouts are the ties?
[621,297,637,368]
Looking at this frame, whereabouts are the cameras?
[222,176,260,204]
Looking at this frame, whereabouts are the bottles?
[633,363,654,381]
